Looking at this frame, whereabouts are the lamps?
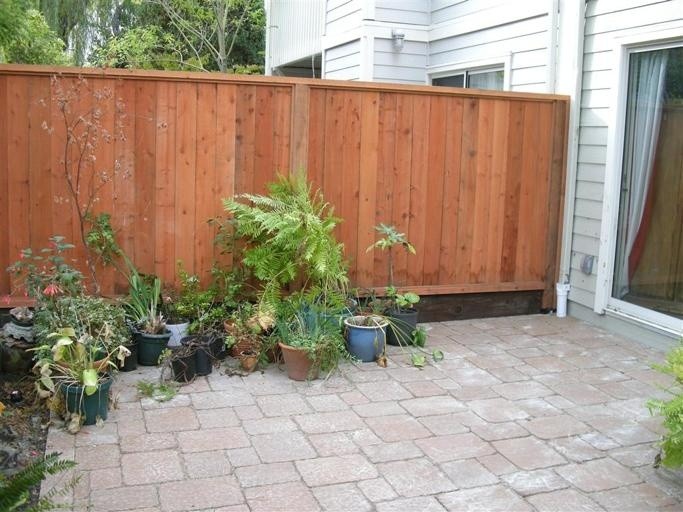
[392,29,405,51]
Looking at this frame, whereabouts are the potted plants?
[4,170,444,437]
